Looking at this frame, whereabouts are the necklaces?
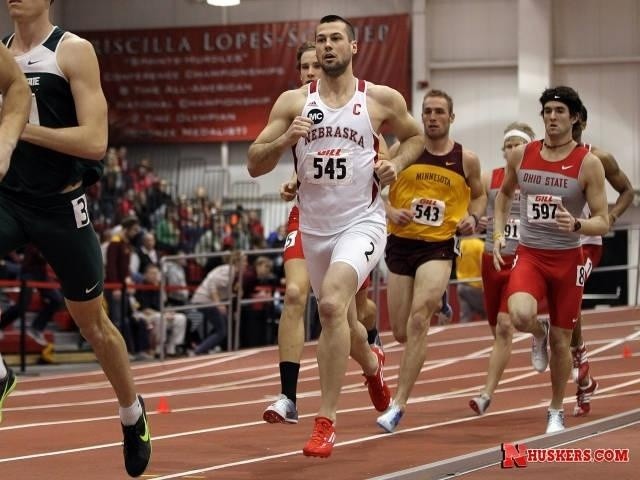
[541,139,577,150]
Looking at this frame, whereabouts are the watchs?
[574,216,581,233]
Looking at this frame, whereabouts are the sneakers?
[302,416,337,458]
[569,336,589,384]
[118,395,153,478]
[262,393,298,425]
[0,358,17,423]
[546,408,566,433]
[573,375,599,417]
[363,345,392,412]
[376,401,403,434]
[530,318,550,374]
[434,289,453,323]
[469,392,491,415]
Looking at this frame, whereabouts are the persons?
[248,13,428,458]
[467,122,536,417]
[262,40,385,425]
[571,105,637,418]
[491,84,612,438]
[0,0,154,476]
[373,89,488,436]
[2,39,34,182]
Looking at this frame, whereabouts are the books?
[1,104,489,364]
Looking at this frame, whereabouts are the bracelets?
[491,233,503,241]
[608,211,619,223]
